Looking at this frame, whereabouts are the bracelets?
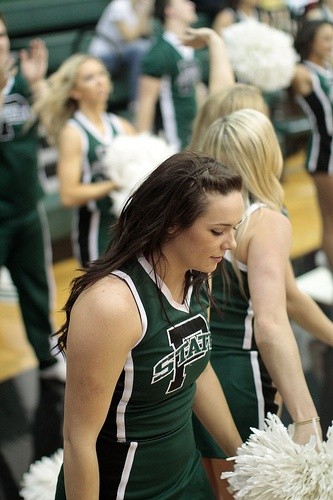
[293,417,320,425]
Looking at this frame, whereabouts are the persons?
[0,1,333,422]
[48,152,246,500]
[190,108,332,499]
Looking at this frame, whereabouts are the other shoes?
[38,360,66,383]
